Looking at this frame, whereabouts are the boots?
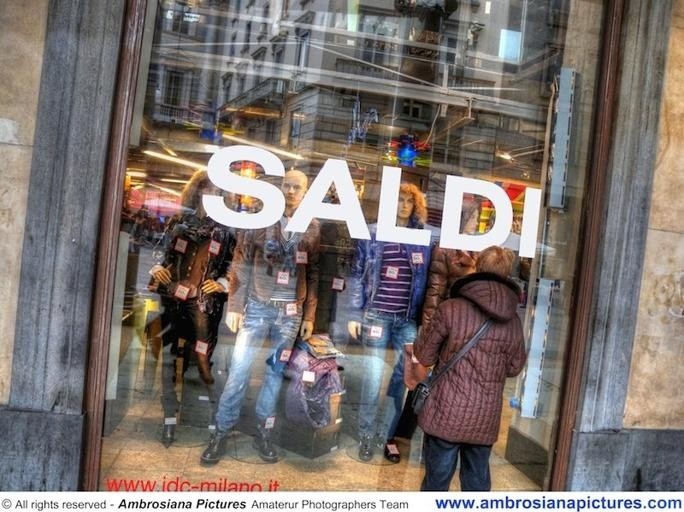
[200,433,226,465]
[253,429,278,461]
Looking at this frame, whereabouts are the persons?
[417,193,484,473]
[117,191,350,386]
[344,180,435,466]
[142,165,238,450]
[199,168,325,471]
[410,243,527,492]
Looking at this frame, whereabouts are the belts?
[247,291,296,309]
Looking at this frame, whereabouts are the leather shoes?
[359,443,374,462]
[162,424,176,449]
[382,444,400,462]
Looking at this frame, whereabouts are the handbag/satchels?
[410,381,431,414]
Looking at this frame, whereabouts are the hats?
[181,168,235,209]
